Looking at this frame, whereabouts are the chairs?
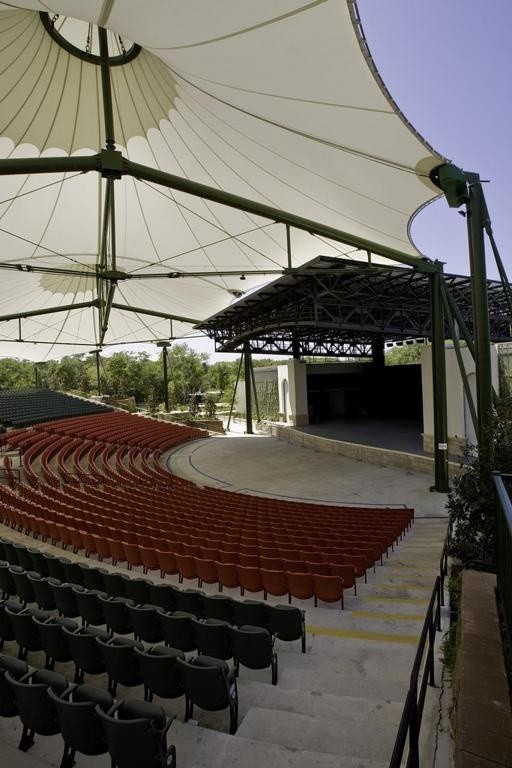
[1,390,416,609]
[0,535,306,767]
